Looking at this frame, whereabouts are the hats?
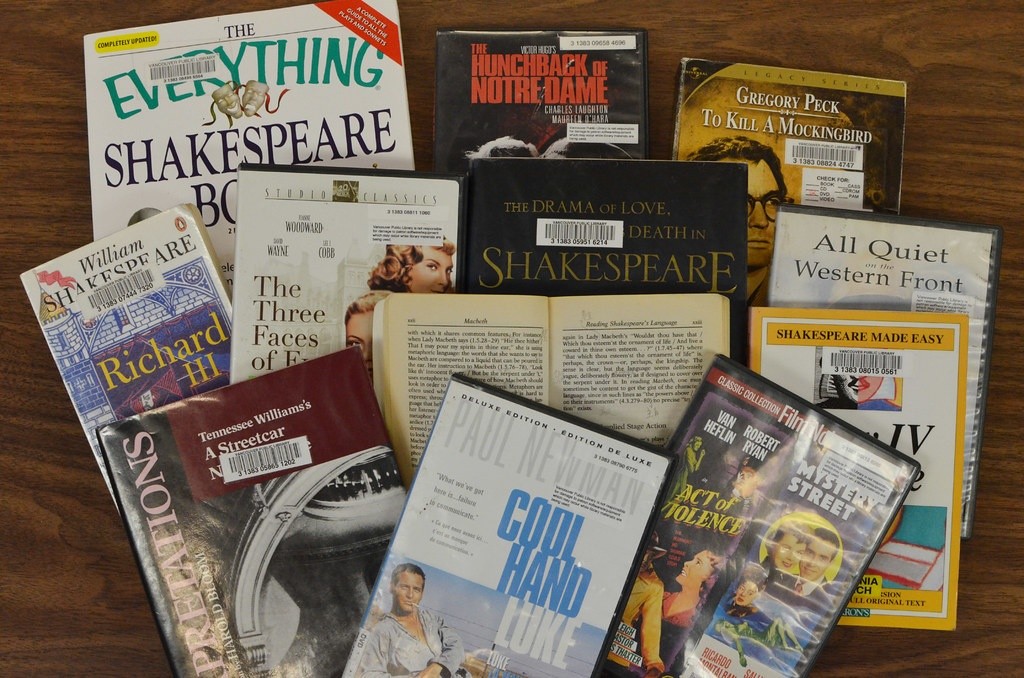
[742,456,763,471]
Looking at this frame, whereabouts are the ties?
[795,579,807,593]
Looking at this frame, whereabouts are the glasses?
[746,192,795,223]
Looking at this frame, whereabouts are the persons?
[345,290,394,386]
[368,235,454,293]
[359,563,473,678]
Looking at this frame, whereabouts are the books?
[19,0,1002,678]
